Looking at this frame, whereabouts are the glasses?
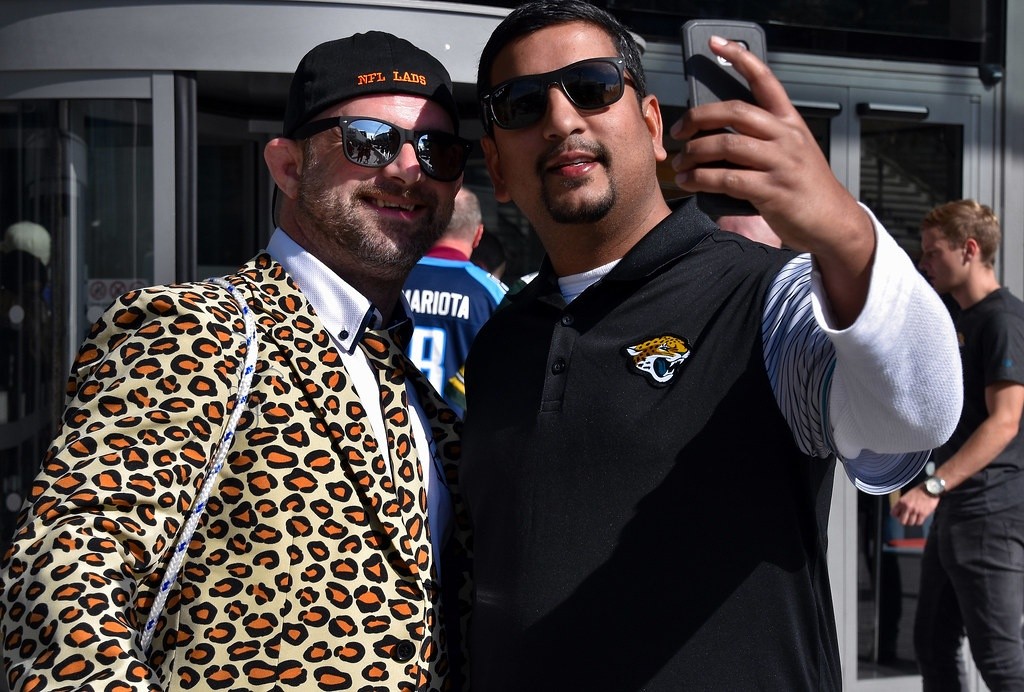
[291,115,473,183]
[482,56,644,145]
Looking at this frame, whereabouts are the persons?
[0,29,470,692]
[890,194,1024,692]
[398,182,513,427]
[437,2,968,692]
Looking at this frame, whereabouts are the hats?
[282,30,459,137]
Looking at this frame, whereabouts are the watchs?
[922,476,948,501]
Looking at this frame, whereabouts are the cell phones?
[680,20,767,215]
